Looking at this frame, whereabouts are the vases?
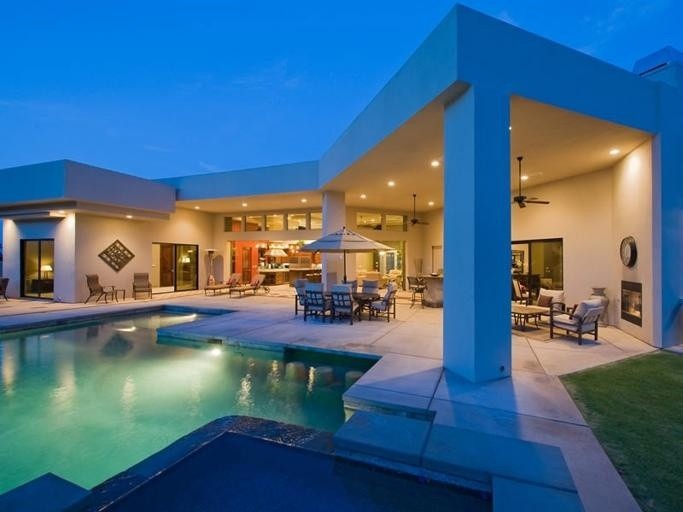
[589,285,608,327]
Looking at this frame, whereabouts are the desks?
[258,267,322,286]
[103,288,126,303]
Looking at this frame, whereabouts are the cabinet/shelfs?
[30,279,54,294]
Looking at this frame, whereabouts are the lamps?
[40,265,53,278]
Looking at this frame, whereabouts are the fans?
[401,191,431,229]
[511,156,551,210]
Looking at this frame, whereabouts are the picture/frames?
[510,248,524,273]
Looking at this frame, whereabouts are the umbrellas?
[263,248,289,263]
[297,226,395,284]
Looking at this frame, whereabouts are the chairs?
[84,273,119,304]
[292,270,442,325]
[510,268,605,346]
[85,324,98,341]
[0,275,9,303]
[132,272,153,299]
[204,271,266,299]
